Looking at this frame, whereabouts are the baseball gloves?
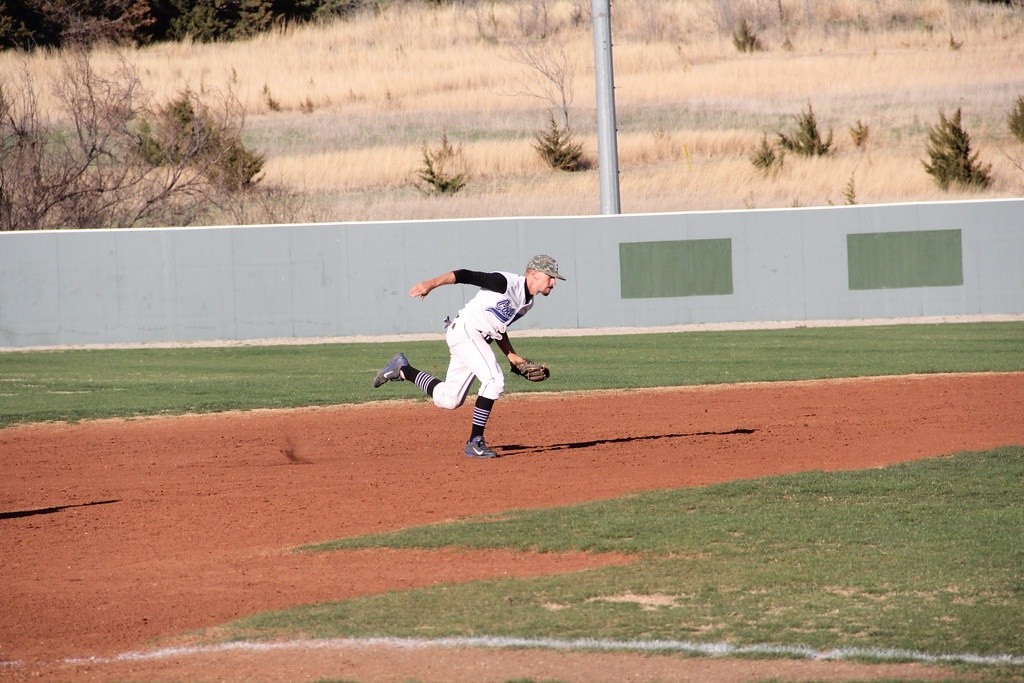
[510,358,550,382]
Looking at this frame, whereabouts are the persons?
[373,254,566,458]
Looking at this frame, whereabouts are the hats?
[527,254,567,281]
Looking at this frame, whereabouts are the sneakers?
[373,352,410,388]
[465,436,497,459]
